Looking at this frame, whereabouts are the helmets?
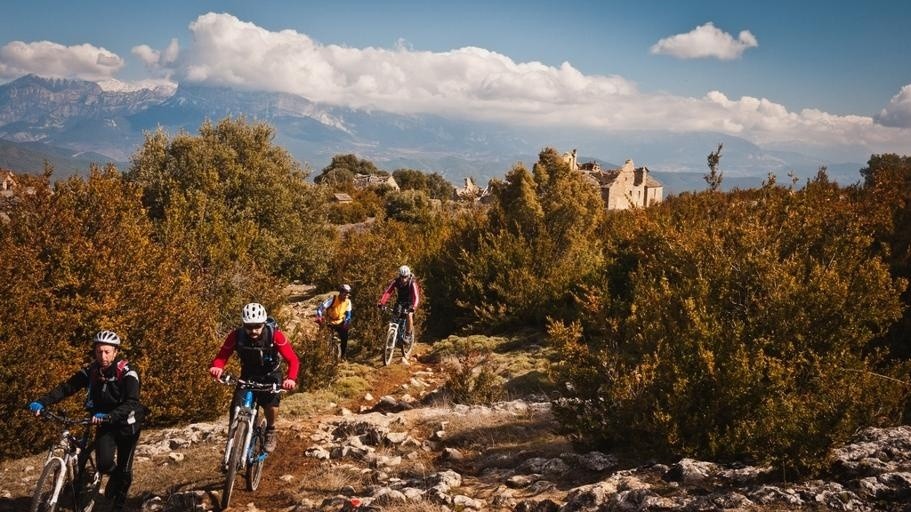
[243,303,267,324]
[93,330,121,346]
[340,284,351,292]
[400,265,410,276]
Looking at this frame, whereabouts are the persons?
[378,265,420,351]
[317,283,353,363]
[26,328,151,509]
[208,303,301,453]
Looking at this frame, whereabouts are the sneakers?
[264,427,277,452]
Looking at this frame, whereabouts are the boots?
[105,462,128,512]
[403,332,412,344]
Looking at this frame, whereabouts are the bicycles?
[218,374,289,509]
[319,320,344,361]
[29,408,107,512]
[376,305,416,366]
